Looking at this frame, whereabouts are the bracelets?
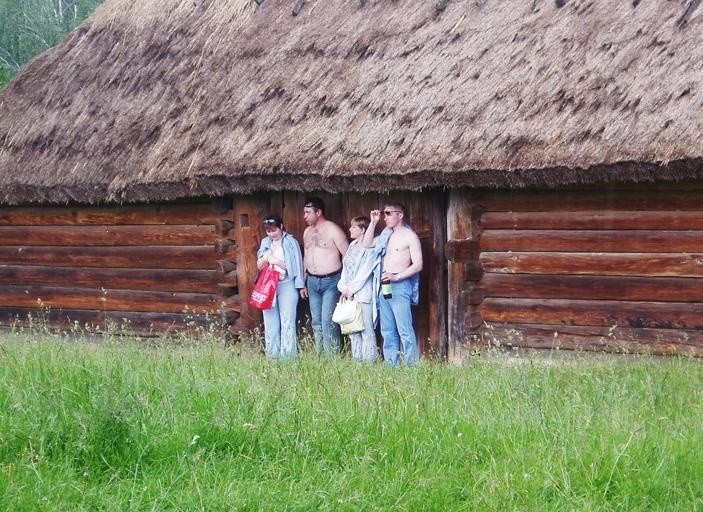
[397,274,401,282]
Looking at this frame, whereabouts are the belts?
[306,270,340,279]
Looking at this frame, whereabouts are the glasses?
[382,210,400,216]
[263,219,278,224]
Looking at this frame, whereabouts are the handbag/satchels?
[337,304,365,335]
[332,294,356,324]
[246,264,280,311]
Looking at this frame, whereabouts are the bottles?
[380,271,392,299]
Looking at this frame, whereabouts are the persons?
[333,214,381,362]
[256,213,306,360]
[360,198,424,370]
[298,196,350,359]
